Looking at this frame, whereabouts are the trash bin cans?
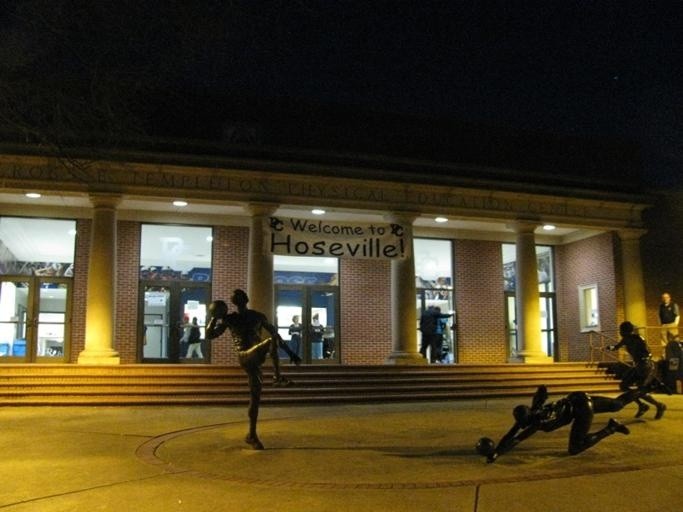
[665,341,683,394]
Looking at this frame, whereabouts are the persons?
[288,314,305,364]
[178,316,192,360]
[184,317,204,360]
[482,377,669,464]
[205,289,305,450]
[655,291,682,358]
[311,314,324,357]
[420,304,456,364]
[603,321,667,419]
